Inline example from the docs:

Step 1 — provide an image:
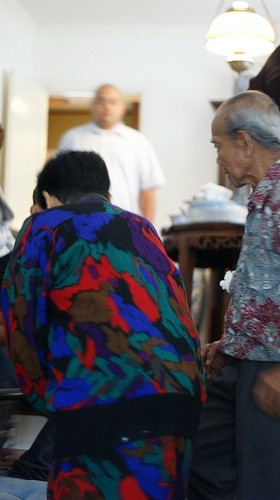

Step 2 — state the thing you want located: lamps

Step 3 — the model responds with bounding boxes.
[204,2,276,74]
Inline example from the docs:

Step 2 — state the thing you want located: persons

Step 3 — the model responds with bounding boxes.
[198,90,279,500]
[56,83,165,224]
[0,187,16,258]
[0,151,208,500]
[30,187,48,215]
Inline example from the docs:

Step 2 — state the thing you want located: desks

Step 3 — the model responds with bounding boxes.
[161,221,243,343]
